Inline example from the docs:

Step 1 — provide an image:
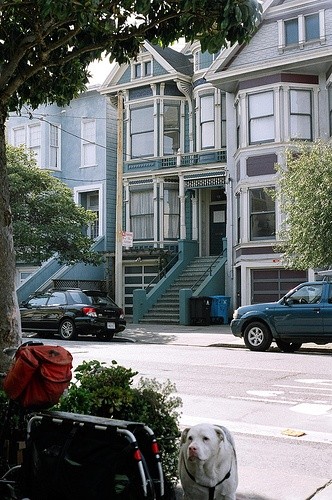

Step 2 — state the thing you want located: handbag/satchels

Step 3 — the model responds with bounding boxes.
[4,343,73,410]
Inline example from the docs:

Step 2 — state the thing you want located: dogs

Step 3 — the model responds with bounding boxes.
[178,424,239,500]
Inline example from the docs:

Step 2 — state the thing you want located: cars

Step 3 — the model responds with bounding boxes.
[230,281,332,353]
[19,287,127,342]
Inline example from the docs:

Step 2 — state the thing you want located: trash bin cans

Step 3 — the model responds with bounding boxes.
[221,237,228,257]
[187,294,232,326]
